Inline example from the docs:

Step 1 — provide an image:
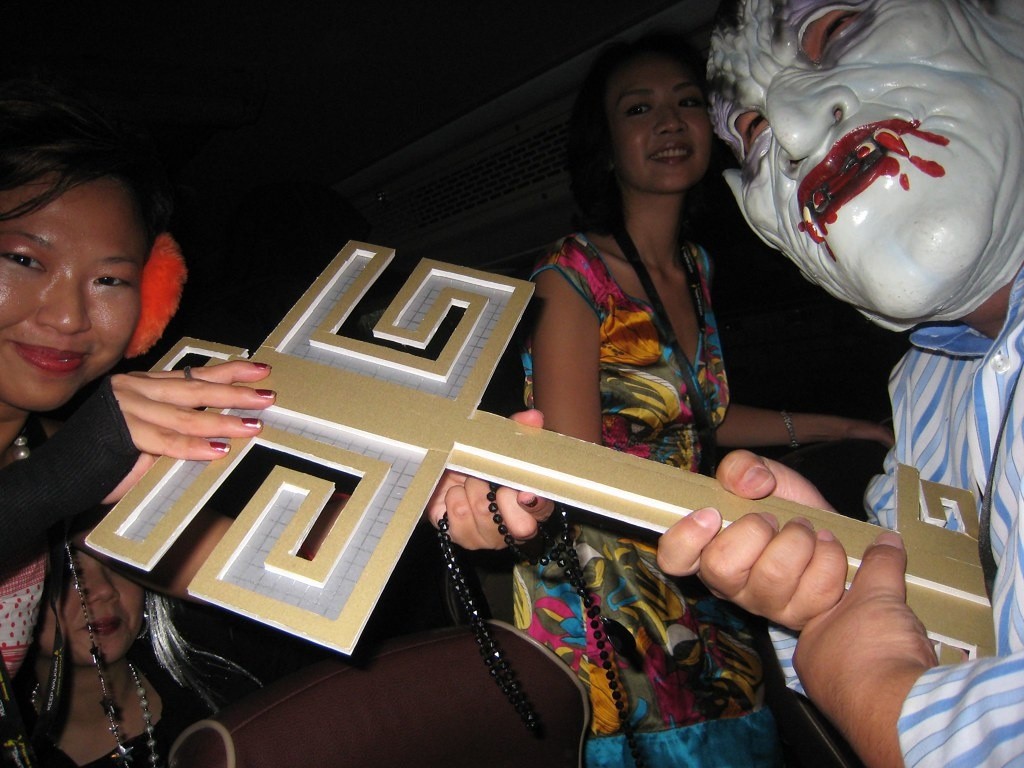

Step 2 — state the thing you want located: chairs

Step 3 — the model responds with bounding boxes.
[169,618,590,768]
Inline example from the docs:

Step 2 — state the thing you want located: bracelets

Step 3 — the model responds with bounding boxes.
[779,411,797,447]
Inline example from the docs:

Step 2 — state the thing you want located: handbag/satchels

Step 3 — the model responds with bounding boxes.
[168,619,591,768]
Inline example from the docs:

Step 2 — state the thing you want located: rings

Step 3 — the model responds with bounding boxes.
[183,365,197,381]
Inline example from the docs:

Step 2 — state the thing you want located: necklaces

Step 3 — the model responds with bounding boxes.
[436,485,646,768]
[13,428,159,767]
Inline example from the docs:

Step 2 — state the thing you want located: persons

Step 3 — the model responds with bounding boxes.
[0,0,1024,768]
